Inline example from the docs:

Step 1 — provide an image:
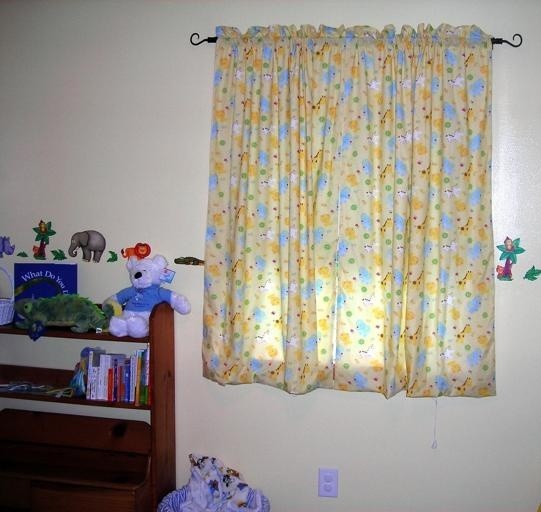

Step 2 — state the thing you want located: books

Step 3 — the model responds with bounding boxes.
[46,342,150,407]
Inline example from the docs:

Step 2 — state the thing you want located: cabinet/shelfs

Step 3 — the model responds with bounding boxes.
[0,301,178,512]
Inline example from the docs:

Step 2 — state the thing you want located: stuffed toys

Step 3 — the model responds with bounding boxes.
[103,255,191,339]
[12,292,123,336]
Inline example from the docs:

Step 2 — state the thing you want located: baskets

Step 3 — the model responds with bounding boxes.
[0,266,16,325]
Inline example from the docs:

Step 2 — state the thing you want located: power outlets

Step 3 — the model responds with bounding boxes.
[318,468,338,498]
